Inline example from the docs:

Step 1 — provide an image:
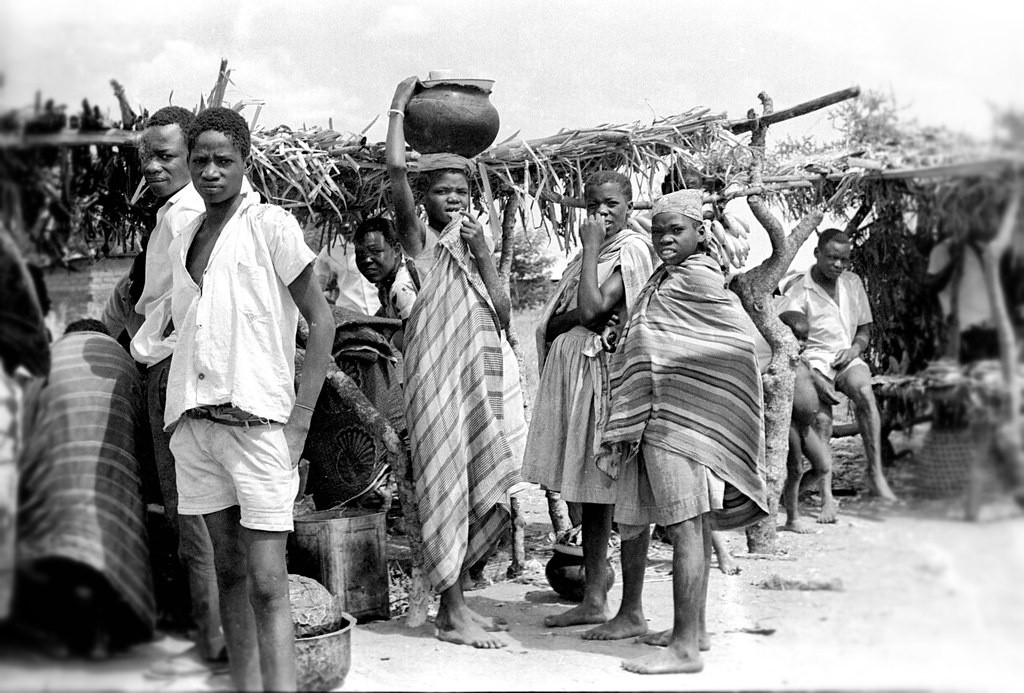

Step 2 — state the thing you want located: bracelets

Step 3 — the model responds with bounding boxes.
[387,109,405,118]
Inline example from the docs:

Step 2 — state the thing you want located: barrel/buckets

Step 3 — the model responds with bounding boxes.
[295,507,392,622]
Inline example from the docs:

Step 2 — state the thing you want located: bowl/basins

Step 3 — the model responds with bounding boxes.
[422,79,495,91]
[555,541,614,560]
[295,612,357,691]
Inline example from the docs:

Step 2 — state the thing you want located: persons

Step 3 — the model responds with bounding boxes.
[0,75,767,693]
[773,229,896,533]
[924,193,1024,365]
